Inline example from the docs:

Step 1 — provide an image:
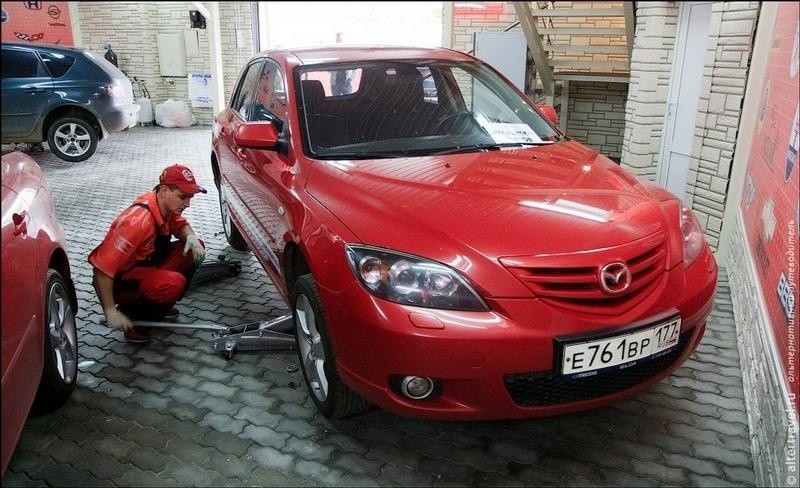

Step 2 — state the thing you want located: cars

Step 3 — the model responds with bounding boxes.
[205,42,720,437]
[416,66,437,96]
[0,41,144,164]
[0,146,83,488]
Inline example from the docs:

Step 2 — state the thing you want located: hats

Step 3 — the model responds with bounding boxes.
[158,162,208,196]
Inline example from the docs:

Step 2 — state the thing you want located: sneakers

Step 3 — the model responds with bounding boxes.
[163,306,181,320]
[123,328,154,343]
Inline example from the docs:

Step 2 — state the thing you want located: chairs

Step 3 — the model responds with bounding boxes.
[296,66,449,148]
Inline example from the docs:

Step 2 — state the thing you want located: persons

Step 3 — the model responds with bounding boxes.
[87,164,209,345]
